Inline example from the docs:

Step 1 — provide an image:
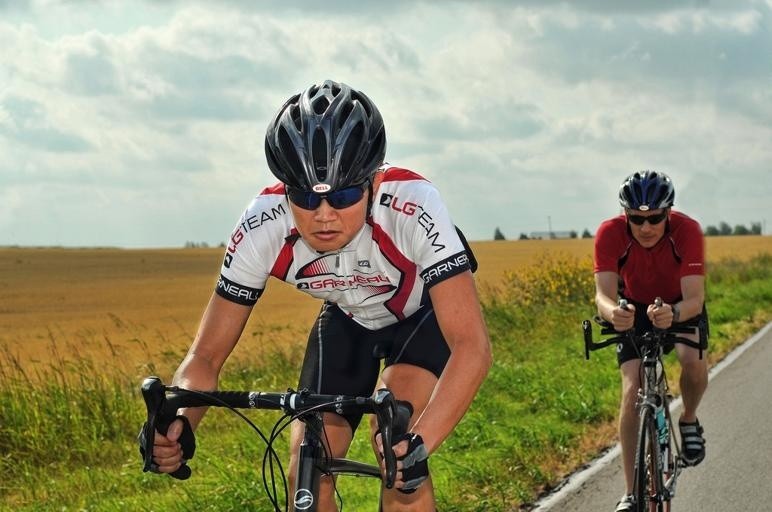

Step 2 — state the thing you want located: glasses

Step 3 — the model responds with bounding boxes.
[282,179,376,210]
[627,208,668,226]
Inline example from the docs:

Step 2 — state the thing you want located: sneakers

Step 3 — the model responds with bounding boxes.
[676,413,707,468]
[612,493,637,511]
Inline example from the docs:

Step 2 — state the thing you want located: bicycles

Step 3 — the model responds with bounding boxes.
[138,373,414,511]
[582,297,705,512]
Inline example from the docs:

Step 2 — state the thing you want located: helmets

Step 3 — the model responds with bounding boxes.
[616,169,676,212]
[263,78,389,195]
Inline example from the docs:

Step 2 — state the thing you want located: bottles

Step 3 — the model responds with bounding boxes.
[657,404,670,444]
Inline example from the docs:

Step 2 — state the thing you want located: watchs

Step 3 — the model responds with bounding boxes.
[670,304,681,320]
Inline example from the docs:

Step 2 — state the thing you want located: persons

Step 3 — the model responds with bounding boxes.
[593,171,709,512]
[139,80,494,511]
